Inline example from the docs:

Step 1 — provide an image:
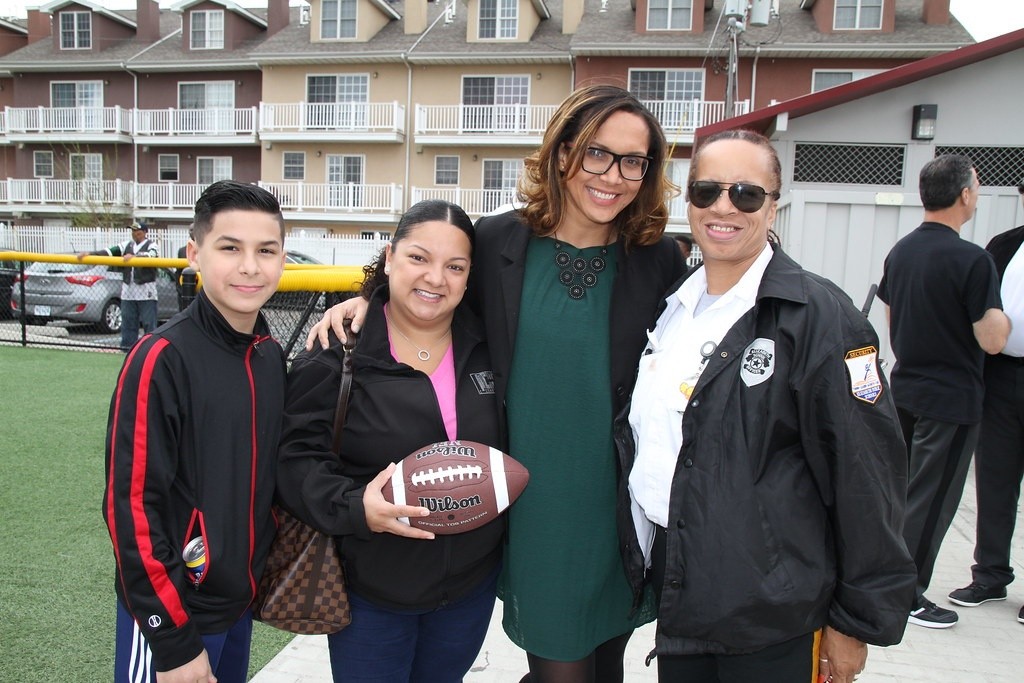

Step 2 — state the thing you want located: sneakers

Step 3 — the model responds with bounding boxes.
[907,595,958,629]
[948,582,1007,606]
[1017,606,1024,623]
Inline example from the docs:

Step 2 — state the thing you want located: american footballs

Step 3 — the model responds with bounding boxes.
[383,440,531,537]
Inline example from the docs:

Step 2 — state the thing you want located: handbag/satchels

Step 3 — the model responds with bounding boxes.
[249,317,351,635]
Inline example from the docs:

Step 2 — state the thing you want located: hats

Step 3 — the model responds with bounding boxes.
[126,222,148,233]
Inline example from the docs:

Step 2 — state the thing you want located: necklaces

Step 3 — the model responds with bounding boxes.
[385,312,452,363]
[548,215,613,301]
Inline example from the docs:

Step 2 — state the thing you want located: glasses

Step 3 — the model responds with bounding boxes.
[687,180,782,213]
[565,142,653,181]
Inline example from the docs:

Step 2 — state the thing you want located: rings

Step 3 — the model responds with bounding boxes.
[819,659,829,663]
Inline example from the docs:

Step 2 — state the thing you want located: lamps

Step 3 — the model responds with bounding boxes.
[473,155,477,160]
[912,104,937,140]
[373,72,377,78]
[105,80,109,84]
[238,80,242,86]
[316,151,321,157]
[537,73,541,79]
[188,153,191,159]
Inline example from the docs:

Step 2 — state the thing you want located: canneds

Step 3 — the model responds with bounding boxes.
[183,536,205,581]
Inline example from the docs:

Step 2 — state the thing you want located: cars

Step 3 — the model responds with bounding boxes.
[176,246,355,312]
[8,250,184,334]
[0,259,37,320]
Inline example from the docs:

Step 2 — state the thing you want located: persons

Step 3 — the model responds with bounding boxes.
[876,154,1012,630]
[300,83,690,683]
[103,181,291,683]
[617,128,918,683]
[948,175,1024,626]
[75,220,161,354]
[173,221,198,317]
[288,202,509,683]
